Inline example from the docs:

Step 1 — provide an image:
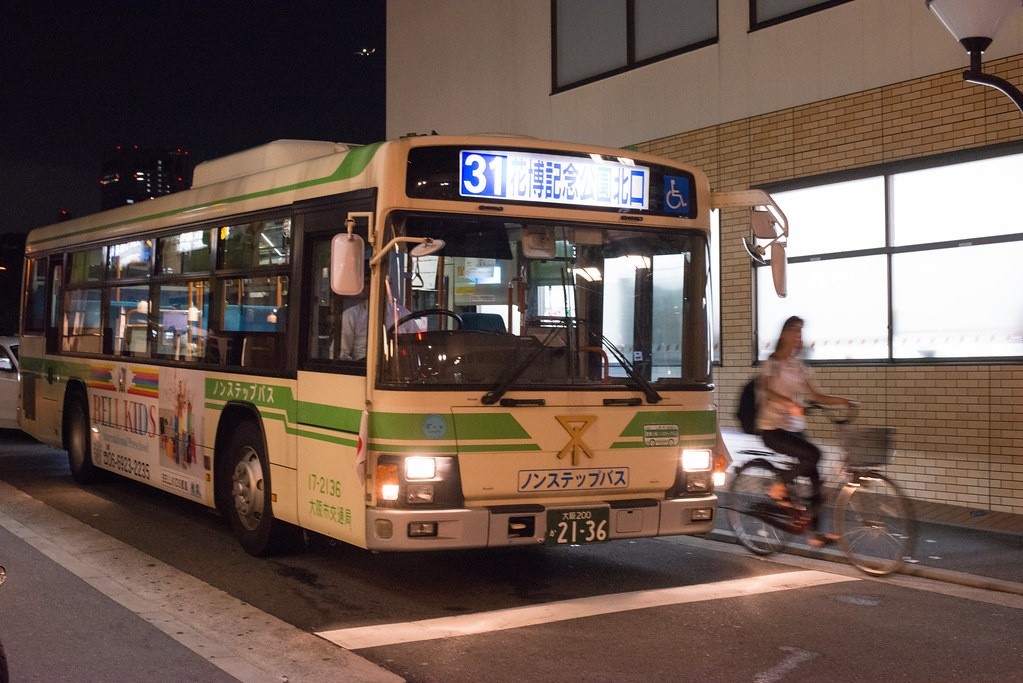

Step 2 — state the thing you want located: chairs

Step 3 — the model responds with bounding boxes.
[458,313,506,333]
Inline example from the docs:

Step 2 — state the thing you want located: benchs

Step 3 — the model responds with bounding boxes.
[206,330,330,370]
[66,327,105,354]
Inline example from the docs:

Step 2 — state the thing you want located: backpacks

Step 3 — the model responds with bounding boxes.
[736,353,783,435]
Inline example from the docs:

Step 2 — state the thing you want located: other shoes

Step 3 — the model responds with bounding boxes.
[808,533,842,548]
[766,487,806,512]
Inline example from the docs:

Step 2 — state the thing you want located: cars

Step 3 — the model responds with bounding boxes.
[0,334,23,429]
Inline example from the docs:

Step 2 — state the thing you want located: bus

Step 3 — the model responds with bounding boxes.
[16,133,788,558]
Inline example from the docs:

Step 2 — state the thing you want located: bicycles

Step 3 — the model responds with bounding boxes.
[717,400,919,577]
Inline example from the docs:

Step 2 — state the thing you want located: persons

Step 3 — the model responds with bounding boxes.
[755,316,860,546]
[188,336,206,356]
[330,259,422,361]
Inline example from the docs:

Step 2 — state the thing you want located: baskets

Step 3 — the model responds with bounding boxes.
[844,427,897,469]
